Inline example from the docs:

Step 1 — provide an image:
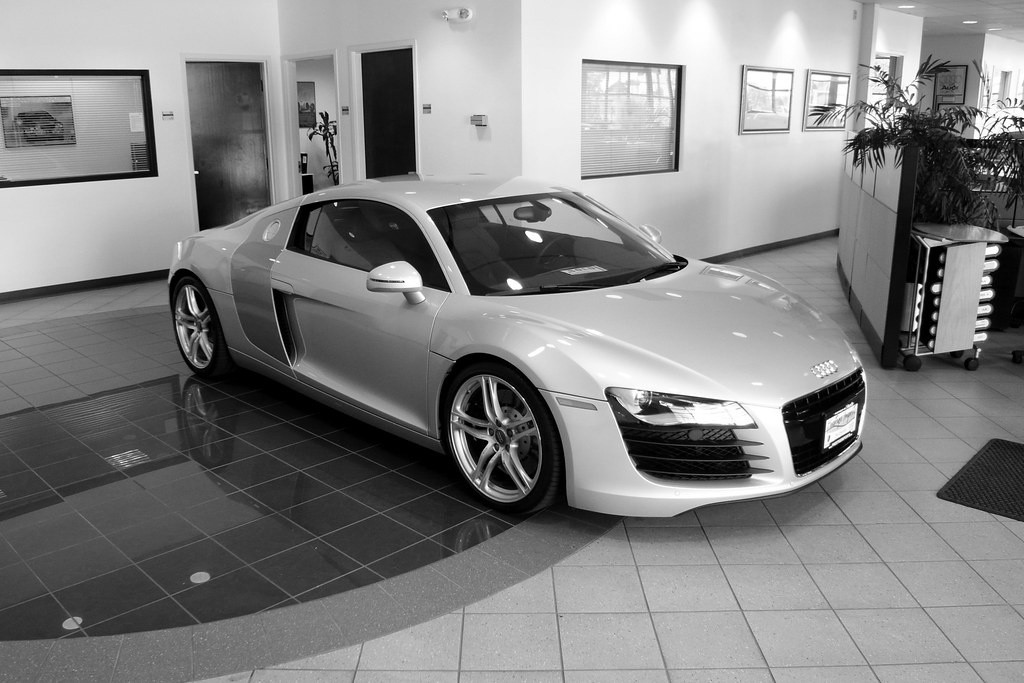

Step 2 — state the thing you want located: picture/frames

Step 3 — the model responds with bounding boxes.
[0,94,76,147]
[802,69,851,132]
[738,64,795,136]
[934,65,968,105]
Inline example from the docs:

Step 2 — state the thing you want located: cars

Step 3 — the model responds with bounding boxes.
[12,111,66,145]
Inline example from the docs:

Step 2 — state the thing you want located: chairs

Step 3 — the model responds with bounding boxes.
[334,206,521,293]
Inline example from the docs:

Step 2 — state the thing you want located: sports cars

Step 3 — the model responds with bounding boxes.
[167,173,867,517]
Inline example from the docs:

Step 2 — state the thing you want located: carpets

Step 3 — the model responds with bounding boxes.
[936,439,1024,521]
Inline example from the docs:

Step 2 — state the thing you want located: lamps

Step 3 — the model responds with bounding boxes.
[441,8,472,21]
